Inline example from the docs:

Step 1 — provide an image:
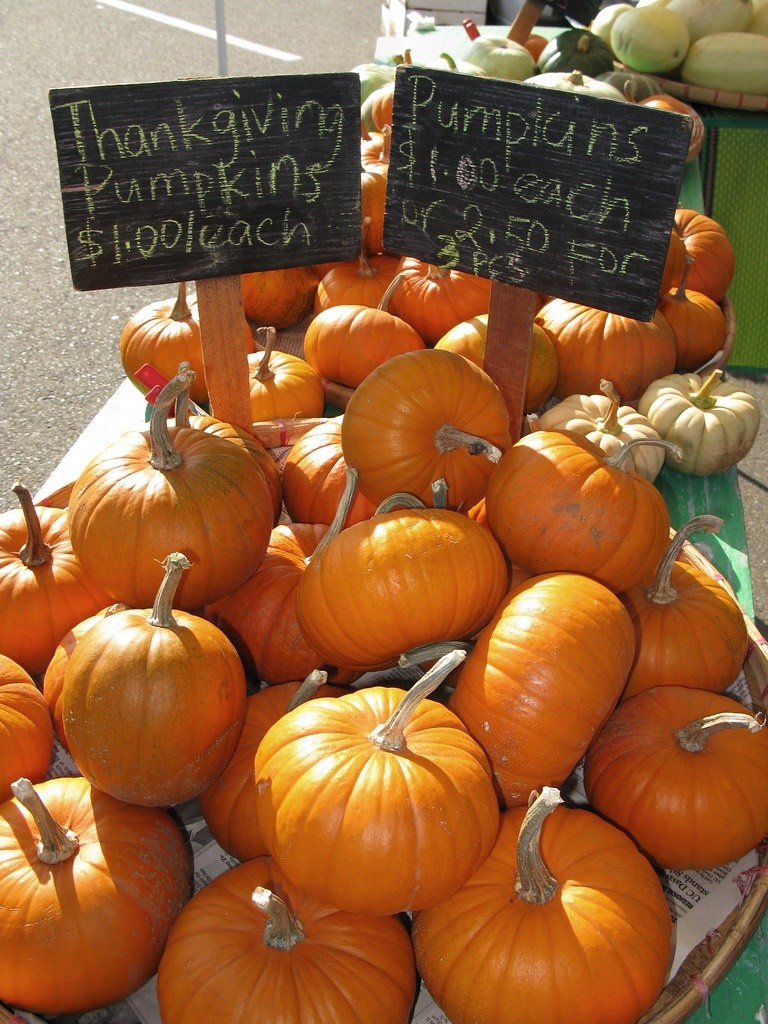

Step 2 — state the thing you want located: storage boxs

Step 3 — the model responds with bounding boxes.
[382,0,487,36]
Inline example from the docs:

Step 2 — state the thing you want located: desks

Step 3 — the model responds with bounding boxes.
[29,26,768,1024]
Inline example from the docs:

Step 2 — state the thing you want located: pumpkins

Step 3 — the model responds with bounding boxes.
[0,0,768,1024]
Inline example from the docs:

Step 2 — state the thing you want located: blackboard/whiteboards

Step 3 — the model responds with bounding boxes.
[380,68,692,323]
[46,73,363,291]
